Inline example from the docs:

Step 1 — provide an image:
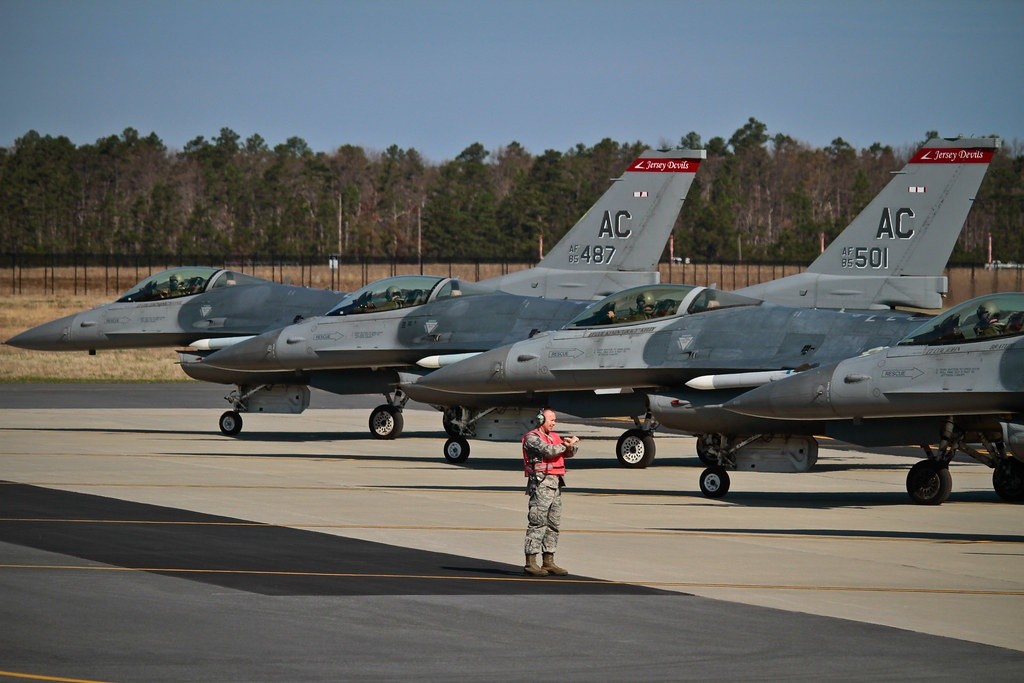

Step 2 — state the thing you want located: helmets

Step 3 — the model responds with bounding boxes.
[169,271,186,290]
[977,301,1001,323]
[385,285,402,302]
[636,292,656,315]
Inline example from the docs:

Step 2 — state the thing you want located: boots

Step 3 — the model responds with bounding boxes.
[541,552,568,576]
[524,553,548,577]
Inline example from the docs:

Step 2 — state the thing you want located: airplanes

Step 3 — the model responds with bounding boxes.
[0,138,1024,507]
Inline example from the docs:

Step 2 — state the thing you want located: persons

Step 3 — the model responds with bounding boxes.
[138,273,190,298]
[352,286,404,313]
[970,300,1006,339]
[522,407,580,577]
[606,291,658,323]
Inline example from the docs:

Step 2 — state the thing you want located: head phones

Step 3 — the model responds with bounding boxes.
[536,408,546,425]
